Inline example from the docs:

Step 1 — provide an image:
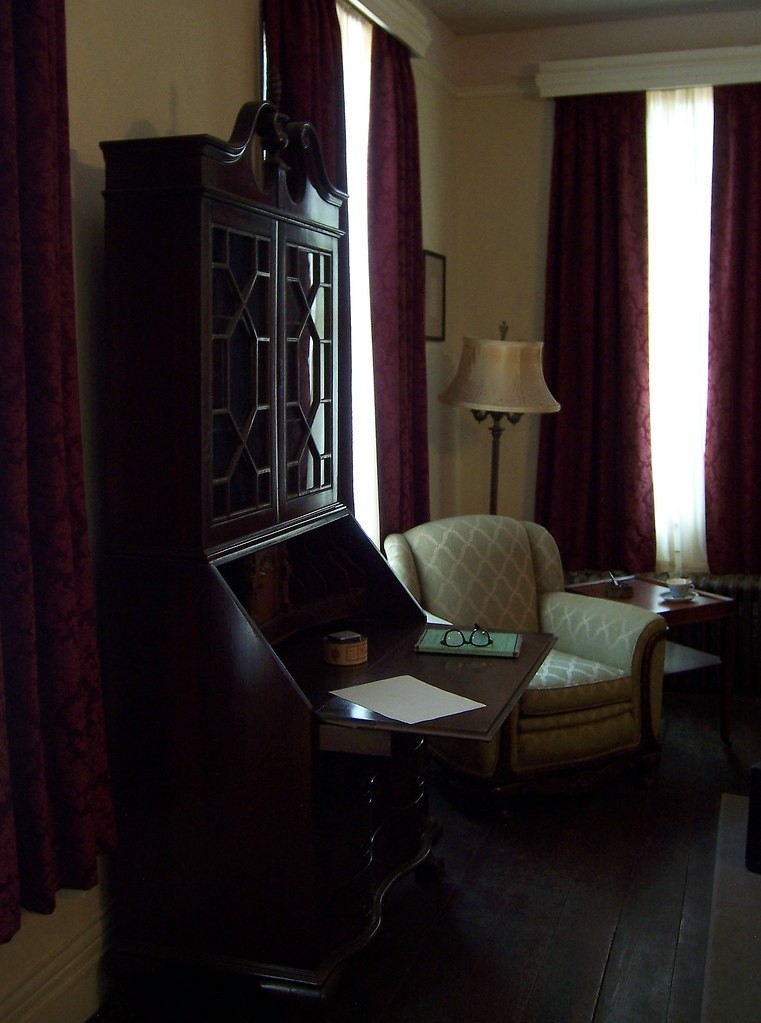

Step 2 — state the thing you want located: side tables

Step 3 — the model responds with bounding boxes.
[566,576,737,751]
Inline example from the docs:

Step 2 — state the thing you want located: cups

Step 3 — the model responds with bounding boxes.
[667,578,694,598]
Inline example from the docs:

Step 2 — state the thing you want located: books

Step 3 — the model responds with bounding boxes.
[414,629,524,657]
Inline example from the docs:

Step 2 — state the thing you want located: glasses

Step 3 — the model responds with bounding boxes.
[440,623,494,646]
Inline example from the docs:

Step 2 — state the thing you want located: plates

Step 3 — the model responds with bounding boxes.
[661,591,698,602]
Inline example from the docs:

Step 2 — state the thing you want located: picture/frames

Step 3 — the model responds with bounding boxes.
[417,249,446,340]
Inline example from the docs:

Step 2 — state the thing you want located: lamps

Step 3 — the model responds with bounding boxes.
[439,317,561,514]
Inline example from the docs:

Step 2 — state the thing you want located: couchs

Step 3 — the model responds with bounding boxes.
[380,513,673,846]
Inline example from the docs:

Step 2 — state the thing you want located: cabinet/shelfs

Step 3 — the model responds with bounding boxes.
[98,101,556,1023]
